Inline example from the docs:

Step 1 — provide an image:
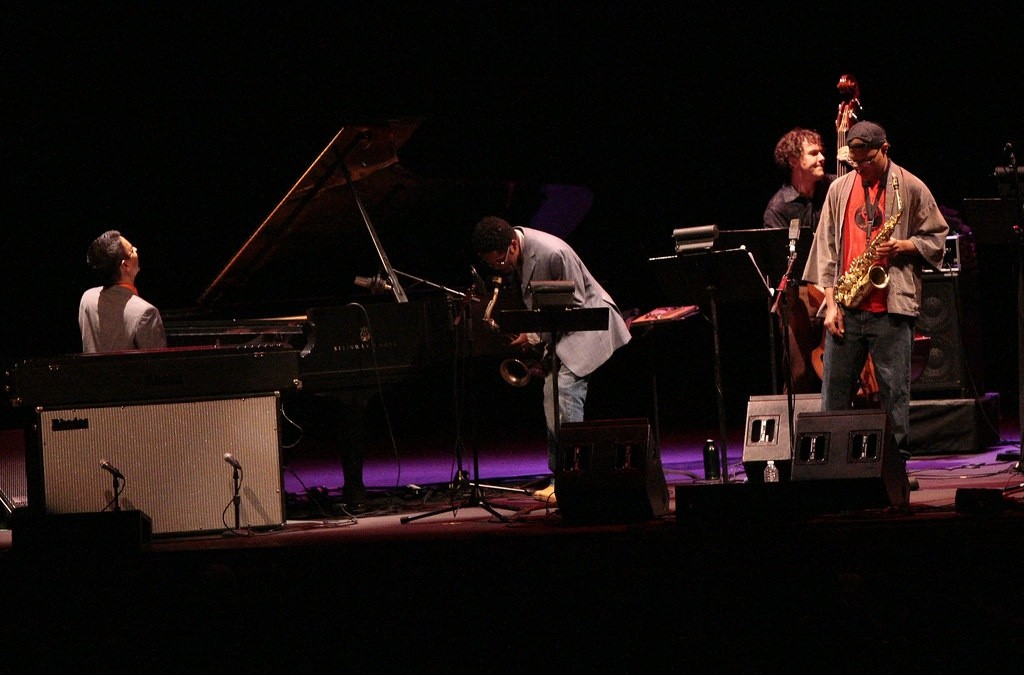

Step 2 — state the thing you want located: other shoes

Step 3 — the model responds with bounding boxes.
[533,482,555,504]
[907,474,920,491]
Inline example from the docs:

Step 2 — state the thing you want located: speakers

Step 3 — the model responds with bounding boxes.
[554,418,670,521]
[908,272,964,391]
[25,390,286,535]
[740,393,910,511]
[12,510,154,549]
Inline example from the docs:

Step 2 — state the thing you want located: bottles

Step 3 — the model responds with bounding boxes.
[764,460,780,482]
[703,439,722,480]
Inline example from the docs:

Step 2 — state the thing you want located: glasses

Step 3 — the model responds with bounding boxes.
[849,143,883,168]
[485,244,511,268]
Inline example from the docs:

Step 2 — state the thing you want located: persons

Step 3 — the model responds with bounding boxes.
[801,122,950,490]
[472,215,632,502]
[764,130,837,394]
[76,230,168,352]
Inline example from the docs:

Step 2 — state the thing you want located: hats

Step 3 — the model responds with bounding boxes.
[846,121,891,148]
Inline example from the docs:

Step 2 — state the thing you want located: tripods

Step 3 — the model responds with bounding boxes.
[381,267,534,525]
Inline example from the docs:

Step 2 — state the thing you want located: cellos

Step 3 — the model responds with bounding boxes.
[809,70,932,398]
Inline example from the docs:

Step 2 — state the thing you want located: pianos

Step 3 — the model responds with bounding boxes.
[3,342,303,512]
[155,124,459,510]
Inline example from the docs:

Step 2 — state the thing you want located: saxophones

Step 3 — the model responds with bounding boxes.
[481,270,548,389]
[833,175,906,308]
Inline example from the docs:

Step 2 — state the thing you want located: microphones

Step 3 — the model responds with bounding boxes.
[223,453,241,469]
[99,459,124,480]
[354,276,392,290]
[789,218,802,252]
[1006,144,1016,164]
